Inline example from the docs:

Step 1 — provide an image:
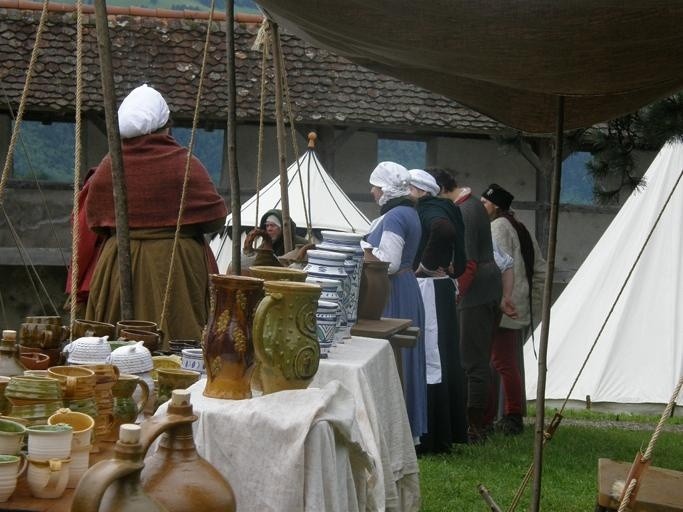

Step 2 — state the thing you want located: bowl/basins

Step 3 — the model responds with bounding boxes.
[16,315,163,348]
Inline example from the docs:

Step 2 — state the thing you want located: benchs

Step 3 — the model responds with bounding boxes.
[598,458,683,512]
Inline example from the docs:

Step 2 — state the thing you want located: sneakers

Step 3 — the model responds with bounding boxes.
[503,413,524,436]
[467,426,487,445]
[493,418,505,432]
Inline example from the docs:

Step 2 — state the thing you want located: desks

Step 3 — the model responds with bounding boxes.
[3,313,389,510]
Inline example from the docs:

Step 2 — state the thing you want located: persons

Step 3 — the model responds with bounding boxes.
[429,172,502,444]
[243,210,309,267]
[88,86,228,346]
[479,185,531,434]
[360,160,424,455]
[405,170,464,456]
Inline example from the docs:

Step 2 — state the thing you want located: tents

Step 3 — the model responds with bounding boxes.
[520,133,683,407]
[208,131,377,282]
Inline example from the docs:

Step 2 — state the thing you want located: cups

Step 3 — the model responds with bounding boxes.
[1,363,150,512]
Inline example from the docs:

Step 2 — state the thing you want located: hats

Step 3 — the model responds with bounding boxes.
[481,183,514,211]
